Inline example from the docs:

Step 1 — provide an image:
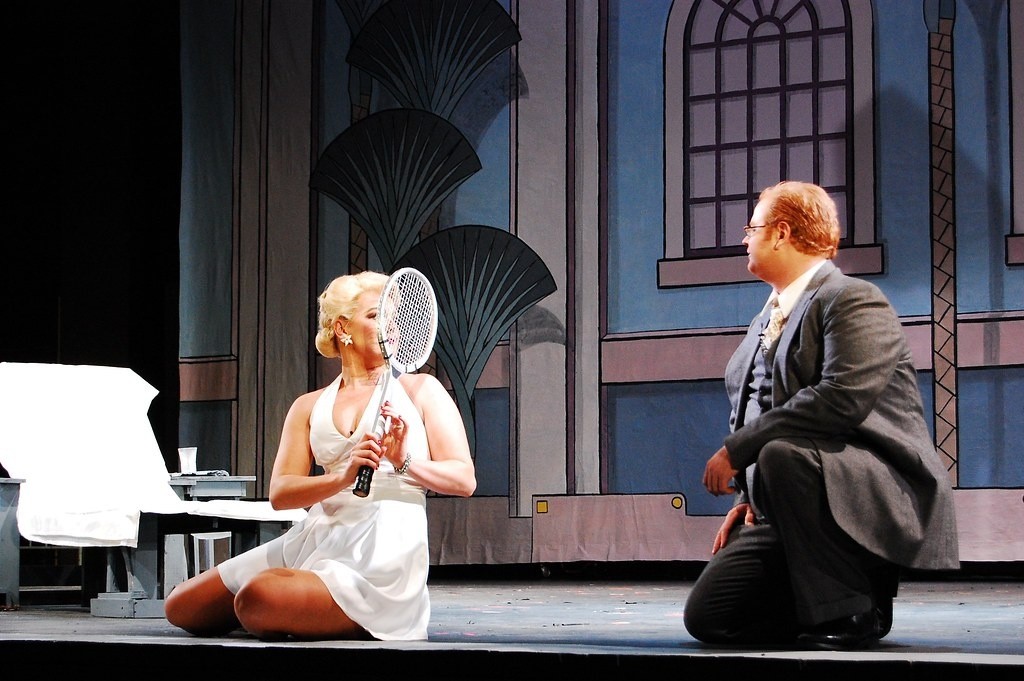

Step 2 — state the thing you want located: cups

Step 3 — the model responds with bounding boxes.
[178,447,197,474]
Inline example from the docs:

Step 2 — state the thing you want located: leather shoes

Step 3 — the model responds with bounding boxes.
[851,567,899,640]
[799,608,877,649]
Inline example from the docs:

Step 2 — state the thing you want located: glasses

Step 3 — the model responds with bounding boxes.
[744,225,765,237]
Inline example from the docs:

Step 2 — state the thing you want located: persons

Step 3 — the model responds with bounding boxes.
[165,271,476,640]
[683,181,961,650]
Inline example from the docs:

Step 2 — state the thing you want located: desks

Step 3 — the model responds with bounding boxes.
[172,472,257,575]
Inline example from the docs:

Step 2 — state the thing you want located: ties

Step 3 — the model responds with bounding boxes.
[761,308,784,349]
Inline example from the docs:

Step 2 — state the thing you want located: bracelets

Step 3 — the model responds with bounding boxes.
[394,452,412,475]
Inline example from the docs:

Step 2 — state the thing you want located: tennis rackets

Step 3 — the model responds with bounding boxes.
[353,265,438,500]
